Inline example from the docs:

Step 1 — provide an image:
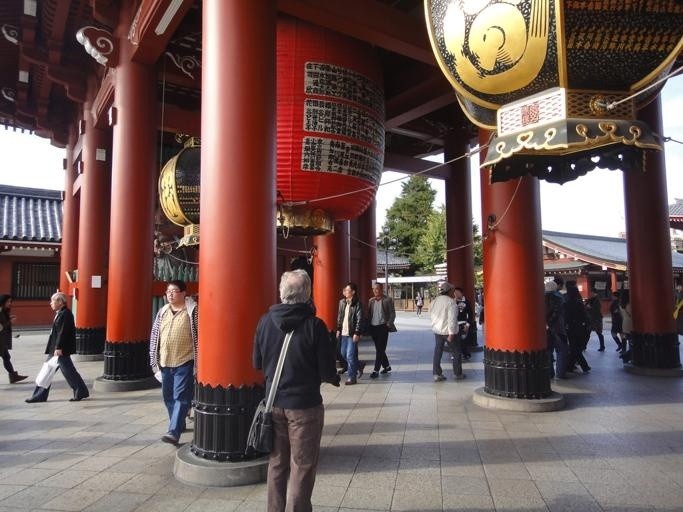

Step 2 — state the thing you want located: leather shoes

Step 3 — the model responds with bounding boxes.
[358,361,366,377]
[70,394,89,401]
[26,399,45,402]
[345,378,356,385]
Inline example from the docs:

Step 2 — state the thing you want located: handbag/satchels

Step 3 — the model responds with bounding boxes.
[245,399,272,455]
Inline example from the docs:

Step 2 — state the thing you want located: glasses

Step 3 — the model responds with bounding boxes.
[166,290,181,294]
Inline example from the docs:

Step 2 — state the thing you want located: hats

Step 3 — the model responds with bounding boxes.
[441,283,454,291]
[545,281,557,292]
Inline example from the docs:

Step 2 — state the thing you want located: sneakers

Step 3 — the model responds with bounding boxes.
[381,367,391,373]
[454,374,466,379]
[162,434,177,444]
[434,374,446,381]
[370,372,378,377]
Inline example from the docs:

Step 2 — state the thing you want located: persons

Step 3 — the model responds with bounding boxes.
[337,287,358,374]
[25,292,89,403]
[0,295,28,383]
[428,283,467,381]
[415,291,424,318]
[335,283,366,385]
[451,287,473,360]
[475,279,683,378]
[149,280,198,445]
[366,283,397,377]
[252,268,341,512]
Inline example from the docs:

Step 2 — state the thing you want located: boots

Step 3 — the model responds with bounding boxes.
[614,336,623,351]
[9,371,27,383]
[619,339,626,353]
[598,335,605,352]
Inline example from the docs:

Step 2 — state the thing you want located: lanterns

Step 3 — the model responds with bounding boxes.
[157,137,201,250]
[276,14,385,236]
[424,0,683,186]
